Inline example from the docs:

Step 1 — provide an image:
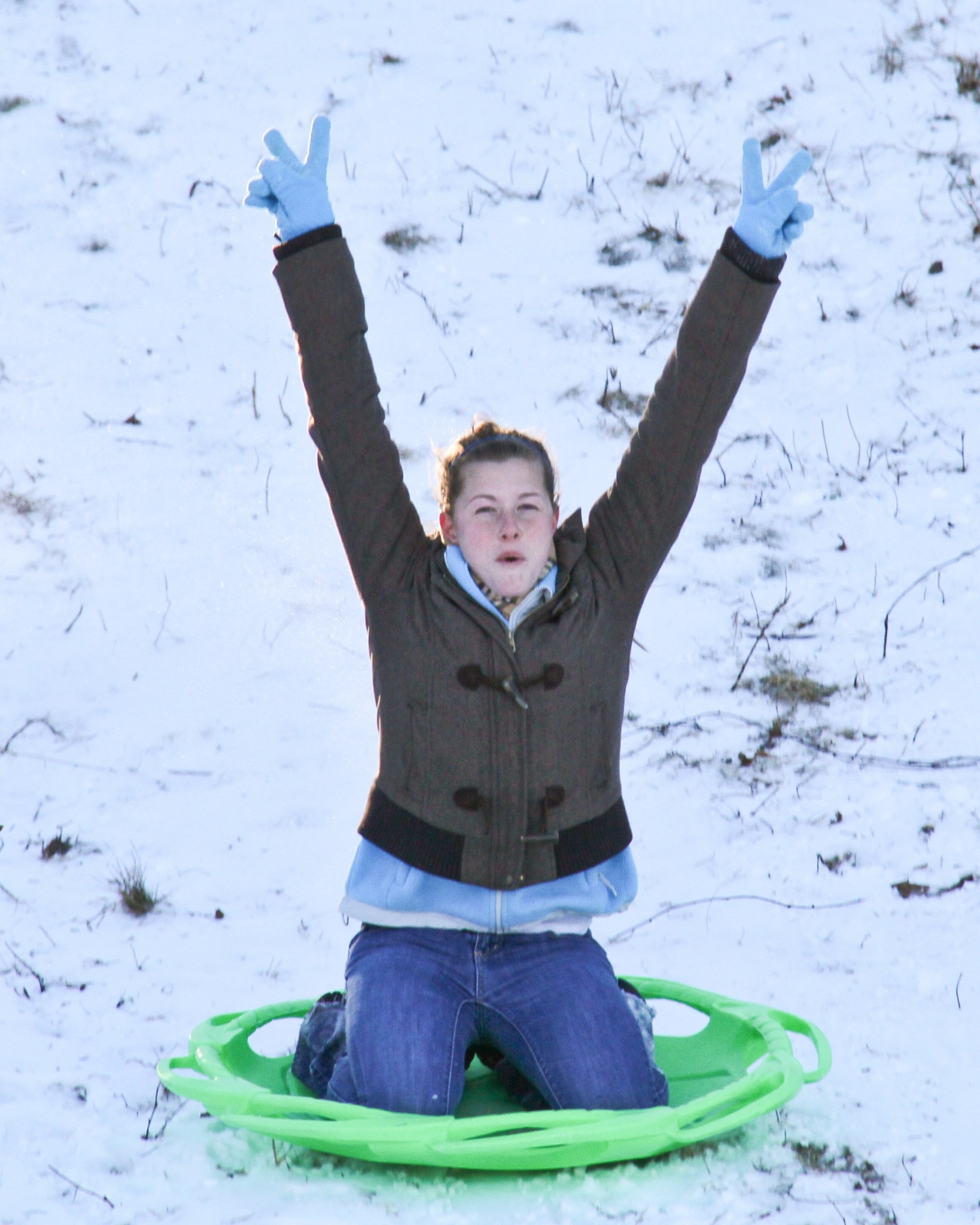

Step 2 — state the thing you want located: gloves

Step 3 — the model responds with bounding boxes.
[242,113,337,239]
[724,135,816,256]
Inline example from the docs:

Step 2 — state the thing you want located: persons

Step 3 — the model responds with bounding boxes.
[242,114,814,1109]
